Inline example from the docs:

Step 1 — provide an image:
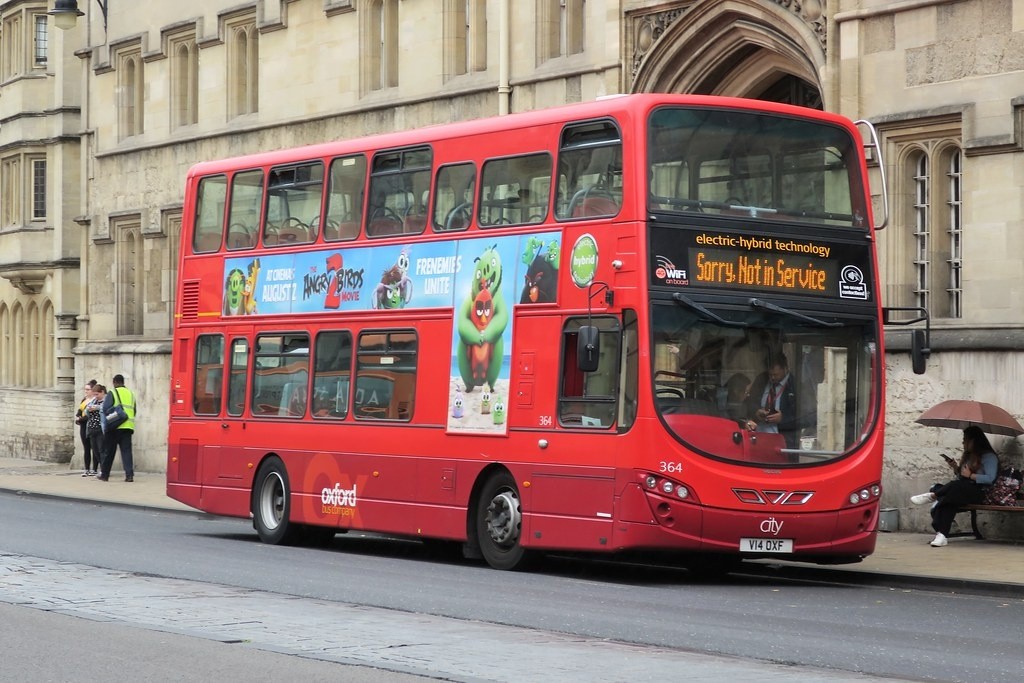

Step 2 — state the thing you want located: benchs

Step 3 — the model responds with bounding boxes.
[949,469,1024,540]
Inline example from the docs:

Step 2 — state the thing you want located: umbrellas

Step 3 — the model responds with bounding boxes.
[915,399,1024,465]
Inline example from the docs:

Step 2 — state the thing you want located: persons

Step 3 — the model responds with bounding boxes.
[911,425,999,547]
[97,374,137,482]
[76,380,108,478]
[703,353,800,464]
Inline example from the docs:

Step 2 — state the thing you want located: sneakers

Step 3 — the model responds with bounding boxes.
[931,532,948,546]
[911,492,935,505]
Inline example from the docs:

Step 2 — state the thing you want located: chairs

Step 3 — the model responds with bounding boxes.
[720,197,748,216]
[196,183,619,251]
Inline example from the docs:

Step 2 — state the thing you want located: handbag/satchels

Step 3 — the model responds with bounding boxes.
[991,467,1024,506]
[105,405,128,431]
[974,484,991,505]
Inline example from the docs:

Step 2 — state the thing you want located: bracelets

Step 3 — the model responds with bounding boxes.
[969,472,973,479]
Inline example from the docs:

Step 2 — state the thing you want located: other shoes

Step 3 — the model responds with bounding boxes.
[88,472,98,475]
[82,470,89,476]
[125,476,134,482]
[96,476,108,482]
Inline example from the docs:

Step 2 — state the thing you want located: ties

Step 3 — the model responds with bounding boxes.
[766,383,778,409]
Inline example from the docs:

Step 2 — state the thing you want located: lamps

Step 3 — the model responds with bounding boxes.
[46,0,107,33]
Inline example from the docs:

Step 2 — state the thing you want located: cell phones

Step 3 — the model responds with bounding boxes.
[940,454,952,462]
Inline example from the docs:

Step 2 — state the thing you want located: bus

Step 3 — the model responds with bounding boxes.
[167,93,935,579]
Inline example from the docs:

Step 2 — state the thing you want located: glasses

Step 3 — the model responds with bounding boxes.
[85,388,92,391]
[744,391,750,395]
